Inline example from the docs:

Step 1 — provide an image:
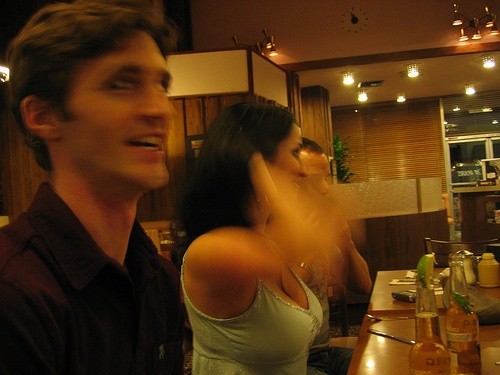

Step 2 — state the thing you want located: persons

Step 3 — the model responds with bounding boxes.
[292,137,372,375]
[0,0,184,375]
[180,103,323,375]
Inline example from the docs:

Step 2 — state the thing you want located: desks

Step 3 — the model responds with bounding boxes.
[349,269,500,375]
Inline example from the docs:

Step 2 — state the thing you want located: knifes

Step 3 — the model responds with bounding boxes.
[367,328,416,345]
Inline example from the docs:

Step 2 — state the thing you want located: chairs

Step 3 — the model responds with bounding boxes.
[425,236,498,267]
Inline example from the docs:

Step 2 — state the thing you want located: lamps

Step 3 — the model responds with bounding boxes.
[231,28,277,57]
[357,90,368,102]
[481,56,495,68]
[407,64,419,77]
[452,3,499,42]
[343,71,354,85]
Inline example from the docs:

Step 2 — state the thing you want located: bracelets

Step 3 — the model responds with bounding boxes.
[297,261,314,285]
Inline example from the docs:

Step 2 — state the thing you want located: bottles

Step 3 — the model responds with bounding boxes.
[455,249,476,285]
[446,255,481,375]
[477,252,500,288]
[409,275,450,375]
